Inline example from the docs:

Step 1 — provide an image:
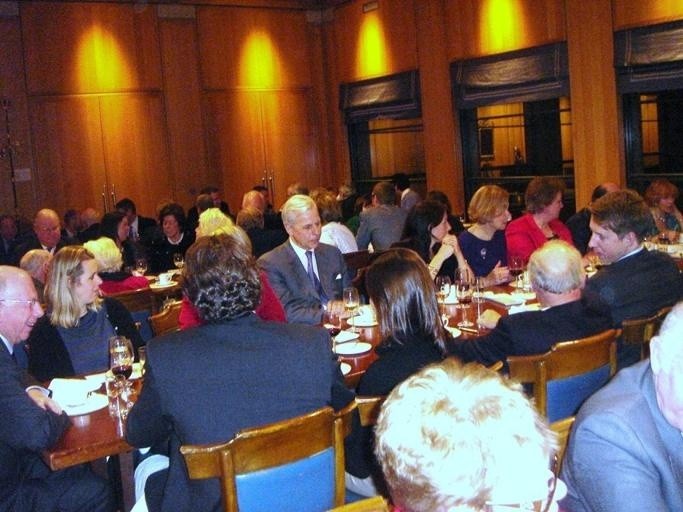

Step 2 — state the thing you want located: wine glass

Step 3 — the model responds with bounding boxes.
[505,253,527,296]
[133,254,185,276]
[326,288,360,341]
[107,334,137,412]
[431,269,488,334]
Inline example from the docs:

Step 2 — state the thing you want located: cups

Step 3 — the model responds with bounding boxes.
[104,375,129,420]
[158,274,172,285]
[137,346,148,366]
[356,304,373,321]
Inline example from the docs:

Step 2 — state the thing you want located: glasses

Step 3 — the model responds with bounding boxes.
[0,298,34,305]
[485,453,558,511]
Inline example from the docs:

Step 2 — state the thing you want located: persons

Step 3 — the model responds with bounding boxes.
[1,174,683,512]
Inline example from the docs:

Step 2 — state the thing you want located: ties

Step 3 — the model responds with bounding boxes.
[130,226,132,232]
[47,247,52,252]
[304,251,321,296]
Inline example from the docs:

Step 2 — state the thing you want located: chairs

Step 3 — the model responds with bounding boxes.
[17,228,683,512]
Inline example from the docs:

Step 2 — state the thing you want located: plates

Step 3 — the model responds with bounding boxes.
[339,362,351,376]
[54,393,110,418]
[148,281,179,288]
[332,339,372,355]
[346,315,378,327]
